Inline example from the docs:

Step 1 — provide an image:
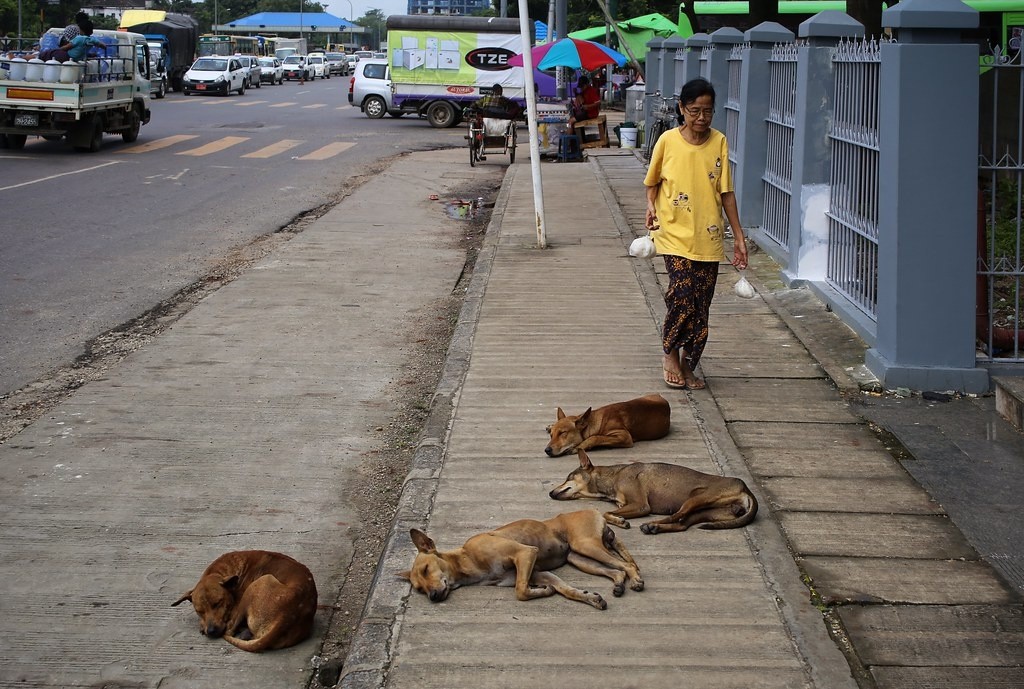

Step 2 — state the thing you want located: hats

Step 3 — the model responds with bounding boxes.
[577,76,590,88]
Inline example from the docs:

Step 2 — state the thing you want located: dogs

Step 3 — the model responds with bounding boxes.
[550,449,758,534]
[545,393,671,457]
[171,550,318,653]
[393,509,644,610]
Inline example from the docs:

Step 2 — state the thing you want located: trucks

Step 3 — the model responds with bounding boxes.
[0,28,167,154]
[119,11,196,93]
[384,12,590,128]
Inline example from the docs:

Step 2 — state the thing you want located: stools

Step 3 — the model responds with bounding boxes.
[558,135,582,163]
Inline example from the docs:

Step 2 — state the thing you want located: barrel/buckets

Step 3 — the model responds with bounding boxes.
[0,52,133,84]
[619,128,638,149]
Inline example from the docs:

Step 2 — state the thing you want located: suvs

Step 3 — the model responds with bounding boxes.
[347,58,421,119]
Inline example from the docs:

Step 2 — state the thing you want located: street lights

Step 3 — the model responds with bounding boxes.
[218,8,230,24]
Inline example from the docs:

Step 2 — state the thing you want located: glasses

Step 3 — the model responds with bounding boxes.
[681,102,715,116]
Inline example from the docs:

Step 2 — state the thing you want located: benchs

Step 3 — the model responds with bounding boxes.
[574,115,611,151]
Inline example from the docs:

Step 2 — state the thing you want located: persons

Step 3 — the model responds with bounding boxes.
[7,20,106,63]
[642,79,749,391]
[472,83,518,128]
[60,11,89,47]
[573,88,579,98]
[32,44,40,51]
[298,57,304,85]
[569,76,601,135]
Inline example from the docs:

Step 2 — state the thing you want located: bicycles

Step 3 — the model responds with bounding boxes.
[644,89,681,166]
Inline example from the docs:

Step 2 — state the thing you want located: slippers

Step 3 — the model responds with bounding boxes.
[678,361,705,390]
[662,357,686,387]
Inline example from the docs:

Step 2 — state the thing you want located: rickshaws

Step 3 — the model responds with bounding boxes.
[461,107,518,167]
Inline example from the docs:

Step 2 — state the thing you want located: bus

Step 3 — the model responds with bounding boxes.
[196,33,259,60]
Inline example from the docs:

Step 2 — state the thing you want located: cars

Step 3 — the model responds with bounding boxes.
[243,36,387,89]
[182,56,246,96]
[146,60,168,98]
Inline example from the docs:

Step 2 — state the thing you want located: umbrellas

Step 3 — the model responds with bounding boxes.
[506,37,629,101]
